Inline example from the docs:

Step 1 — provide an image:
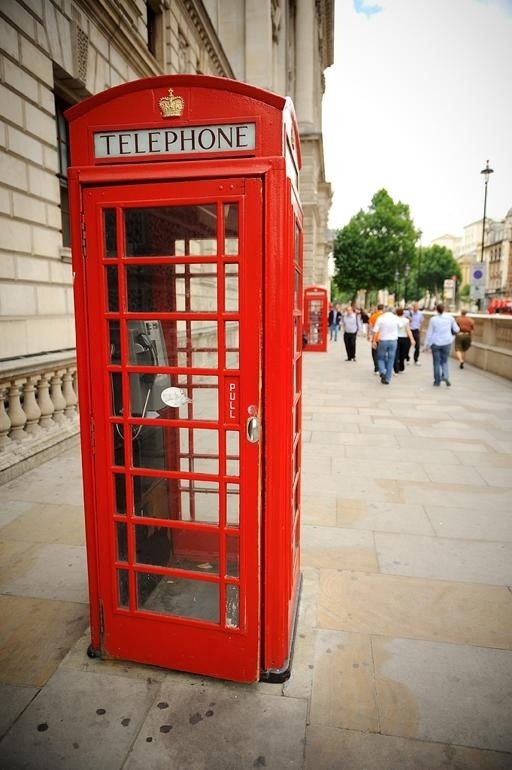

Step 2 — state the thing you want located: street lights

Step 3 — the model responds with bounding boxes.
[394,265,409,309]
[415,229,423,301]
[480,161,494,264]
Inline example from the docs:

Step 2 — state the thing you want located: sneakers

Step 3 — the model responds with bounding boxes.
[329,337,464,386]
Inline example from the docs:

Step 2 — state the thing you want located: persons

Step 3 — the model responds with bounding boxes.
[309,301,475,386]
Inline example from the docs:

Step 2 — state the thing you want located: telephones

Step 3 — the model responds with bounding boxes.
[110,320,172,415]
